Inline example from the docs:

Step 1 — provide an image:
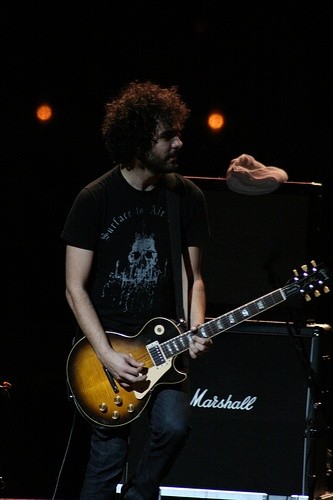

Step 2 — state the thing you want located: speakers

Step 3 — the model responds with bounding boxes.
[158,319,323,496]
[179,176,325,318]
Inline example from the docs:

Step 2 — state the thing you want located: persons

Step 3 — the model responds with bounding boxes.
[51,81,212,500]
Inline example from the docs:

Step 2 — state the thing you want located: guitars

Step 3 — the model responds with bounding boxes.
[64,259,331,430]
